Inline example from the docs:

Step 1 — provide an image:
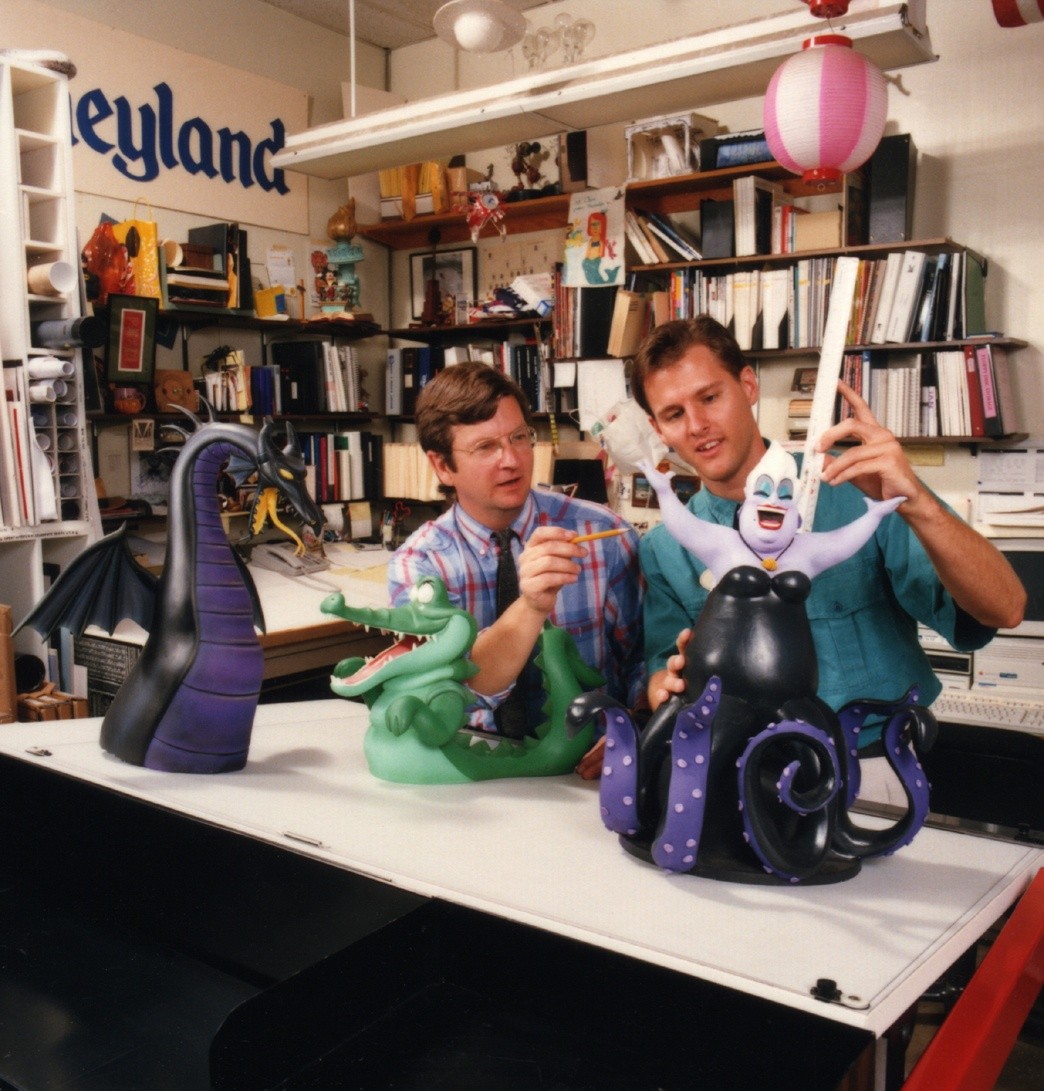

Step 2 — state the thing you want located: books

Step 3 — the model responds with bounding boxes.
[623,165,867,265]
[606,247,985,357]
[782,343,1006,440]
[200,336,361,414]
[0,357,42,531]
[918,492,1044,706]
[161,221,257,318]
[268,427,378,503]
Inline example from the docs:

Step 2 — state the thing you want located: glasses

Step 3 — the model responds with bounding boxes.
[447,426,537,457]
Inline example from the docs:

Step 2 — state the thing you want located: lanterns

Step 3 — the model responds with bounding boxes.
[762,33,889,186]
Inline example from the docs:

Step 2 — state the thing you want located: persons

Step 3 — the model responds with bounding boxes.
[386,357,639,781]
[626,314,1027,814]
[632,438,912,713]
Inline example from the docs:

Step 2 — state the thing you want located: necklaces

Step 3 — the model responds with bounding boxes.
[738,531,795,572]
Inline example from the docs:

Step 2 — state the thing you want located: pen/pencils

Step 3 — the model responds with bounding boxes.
[571,528,631,543]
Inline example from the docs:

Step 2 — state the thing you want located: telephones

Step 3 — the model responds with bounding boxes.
[250,541,329,576]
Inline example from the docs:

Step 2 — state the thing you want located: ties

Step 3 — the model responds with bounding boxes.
[491,530,536,737]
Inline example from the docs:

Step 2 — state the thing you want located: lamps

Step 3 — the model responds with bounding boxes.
[431,0,528,50]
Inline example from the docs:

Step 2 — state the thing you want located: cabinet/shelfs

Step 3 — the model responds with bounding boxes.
[343,133,1044,751]
[93,285,377,553]
[1,49,103,723]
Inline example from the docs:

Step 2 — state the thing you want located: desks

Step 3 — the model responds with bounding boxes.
[77,545,399,717]
